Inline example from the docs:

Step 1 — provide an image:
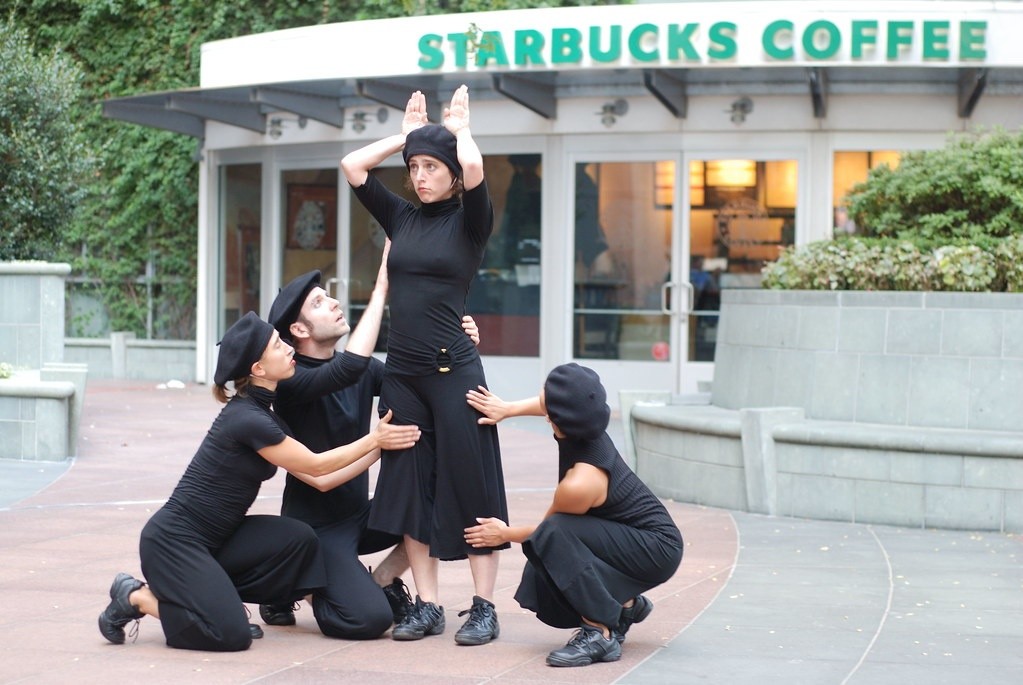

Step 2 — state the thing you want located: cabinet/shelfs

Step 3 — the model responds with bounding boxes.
[710,212,795,272]
[575,280,627,357]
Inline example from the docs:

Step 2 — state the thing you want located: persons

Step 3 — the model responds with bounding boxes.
[97,312,422,653]
[268,233,480,640]
[580,243,620,319]
[665,254,720,310]
[341,85,511,648]
[464,364,683,668]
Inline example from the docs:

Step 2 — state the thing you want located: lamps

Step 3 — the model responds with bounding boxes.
[601,98,628,126]
[270,116,308,137]
[351,106,388,131]
[729,96,753,122]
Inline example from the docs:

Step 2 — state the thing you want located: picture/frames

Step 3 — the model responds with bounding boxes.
[285,183,337,249]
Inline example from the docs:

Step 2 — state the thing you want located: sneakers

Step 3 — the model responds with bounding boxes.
[259,600,301,625]
[392,595,446,639]
[615,594,653,643]
[455,595,500,645]
[98,572,147,644]
[382,577,416,624]
[546,620,621,667]
[242,604,264,639]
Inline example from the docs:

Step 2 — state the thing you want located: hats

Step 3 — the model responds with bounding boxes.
[214,311,275,386]
[544,362,610,440]
[268,270,324,341]
[402,124,462,181]
